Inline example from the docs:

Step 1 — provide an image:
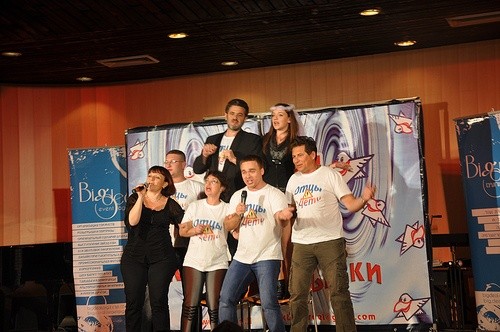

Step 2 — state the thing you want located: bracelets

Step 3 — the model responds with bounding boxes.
[360,195,367,204]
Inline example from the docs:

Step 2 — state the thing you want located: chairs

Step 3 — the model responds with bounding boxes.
[247,286,319,332]
[200,287,250,332]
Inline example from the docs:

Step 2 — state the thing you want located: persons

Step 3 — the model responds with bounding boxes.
[219,154,294,332]
[180,170,239,332]
[119,165,190,332]
[282,135,376,332]
[165,99,306,301]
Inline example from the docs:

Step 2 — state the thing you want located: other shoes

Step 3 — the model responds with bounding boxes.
[276,279,288,300]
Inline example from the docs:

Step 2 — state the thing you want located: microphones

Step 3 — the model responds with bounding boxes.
[132,183,149,194]
[240,191,247,219]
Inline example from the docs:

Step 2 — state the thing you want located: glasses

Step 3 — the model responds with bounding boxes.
[164,159,184,164]
